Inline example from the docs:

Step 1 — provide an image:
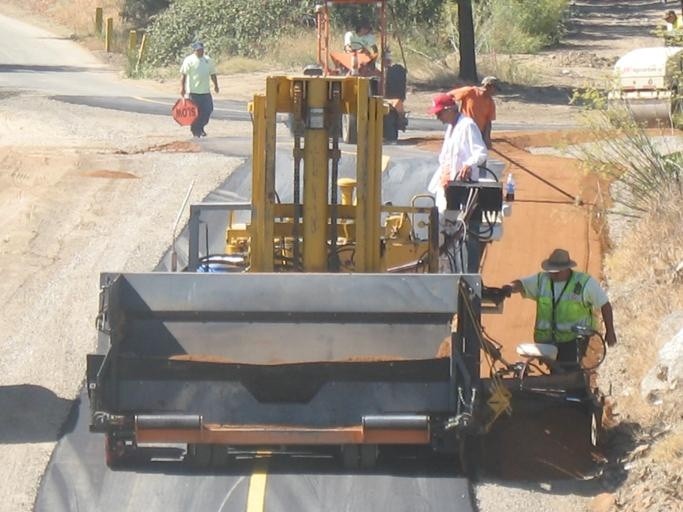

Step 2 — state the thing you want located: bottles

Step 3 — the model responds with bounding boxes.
[481,75,497,86]
[426,93,456,114]
[541,248,578,272]
[191,42,203,50]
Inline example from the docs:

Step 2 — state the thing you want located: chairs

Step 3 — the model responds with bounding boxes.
[200,129,206,136]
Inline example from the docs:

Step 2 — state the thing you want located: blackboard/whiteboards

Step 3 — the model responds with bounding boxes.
[286,0,408,144]
[85,74,510,466]
[603,44,683,129]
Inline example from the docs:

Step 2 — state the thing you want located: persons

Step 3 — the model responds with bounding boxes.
[178,42,219,138]
[448,77,502,180]
[344,23,378,75]
[502,249,617,370]
[428,94,487,245]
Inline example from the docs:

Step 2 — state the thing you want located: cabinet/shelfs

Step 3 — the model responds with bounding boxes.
[505,173,515,202]
